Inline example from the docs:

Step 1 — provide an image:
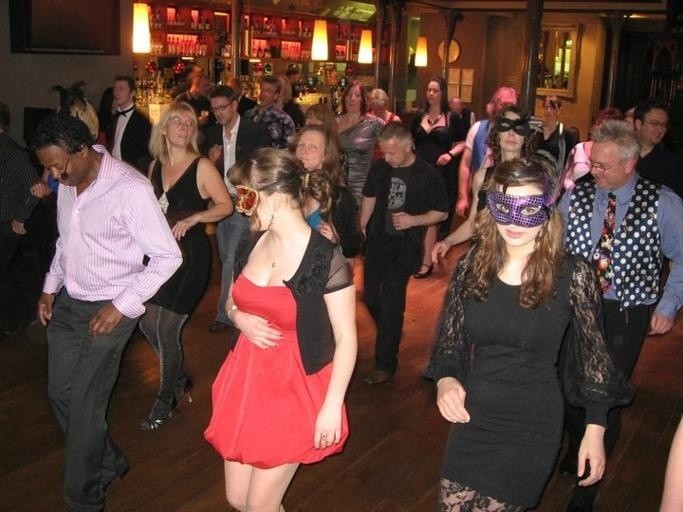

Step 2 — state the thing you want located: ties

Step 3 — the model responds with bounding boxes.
[592,193,616,295]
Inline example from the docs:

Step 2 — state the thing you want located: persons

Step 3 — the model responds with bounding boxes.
[0,60,682,429]
[658,416,682,512]
[550,118,682,512]
[204,148,358,512]
[30,114,182,512]
[418,154,633,512]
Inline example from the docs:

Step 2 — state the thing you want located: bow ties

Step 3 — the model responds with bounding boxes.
[116,110,126,118]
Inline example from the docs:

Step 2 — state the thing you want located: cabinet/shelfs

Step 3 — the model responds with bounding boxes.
[118,0,236,106]
[235,0,376,105]
[6,1,121,59]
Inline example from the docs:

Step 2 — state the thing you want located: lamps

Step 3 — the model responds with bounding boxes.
[413,0,428,67]
[311,0,328,61]
[356,1,374,64]
[130,1,152,53]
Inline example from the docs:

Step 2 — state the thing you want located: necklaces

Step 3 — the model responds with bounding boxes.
[270,258,279,268]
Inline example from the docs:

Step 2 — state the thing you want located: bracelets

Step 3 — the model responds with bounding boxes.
[224,304,236,323]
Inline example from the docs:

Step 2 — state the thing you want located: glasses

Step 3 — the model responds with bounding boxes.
[212,101,232,112]
[585,159,623,172]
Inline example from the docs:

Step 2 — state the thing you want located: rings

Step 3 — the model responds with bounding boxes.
[322,434,325,440]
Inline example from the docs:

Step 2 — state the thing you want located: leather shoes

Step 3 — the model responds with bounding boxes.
[415,261,434,278]
[209,321,228,332]
[364,369,392,383]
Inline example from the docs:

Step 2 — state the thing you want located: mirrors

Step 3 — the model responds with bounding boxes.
[520,21,581,100]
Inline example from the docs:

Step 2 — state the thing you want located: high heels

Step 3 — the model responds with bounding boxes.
[170,376,193,416]
[139,396,176,432]
